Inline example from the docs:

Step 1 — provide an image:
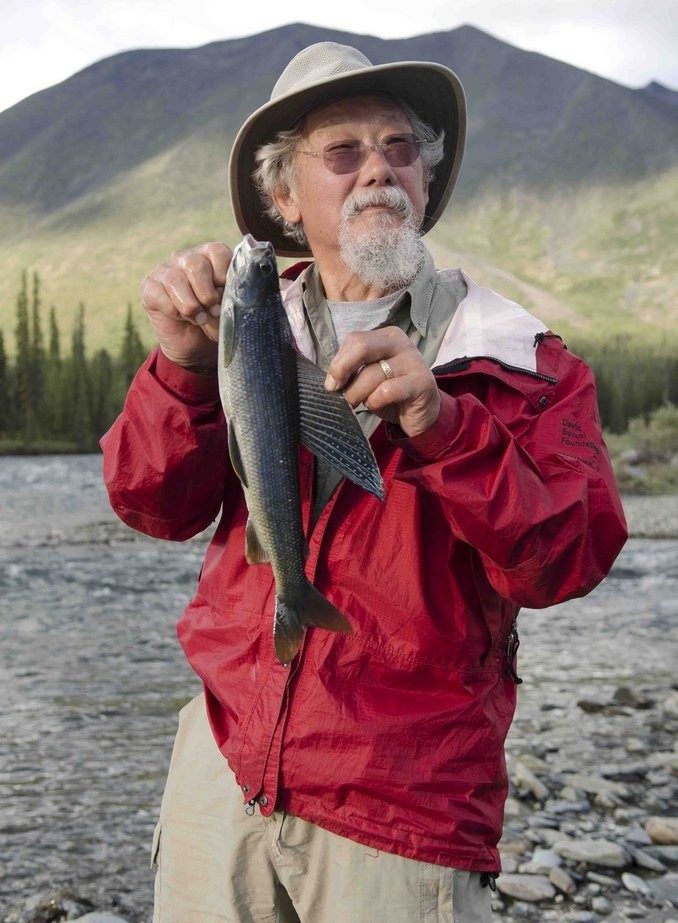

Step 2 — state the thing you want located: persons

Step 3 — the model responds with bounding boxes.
[98,42,629,923]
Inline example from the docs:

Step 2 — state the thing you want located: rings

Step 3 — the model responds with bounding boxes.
[378,359,394,380]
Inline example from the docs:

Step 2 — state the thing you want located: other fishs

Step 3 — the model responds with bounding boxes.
[216,234,386,669]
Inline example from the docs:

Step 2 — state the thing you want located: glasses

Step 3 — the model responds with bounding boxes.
[288,133,425,175]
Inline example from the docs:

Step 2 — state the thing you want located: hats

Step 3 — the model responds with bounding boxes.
[230,41,467,258]
